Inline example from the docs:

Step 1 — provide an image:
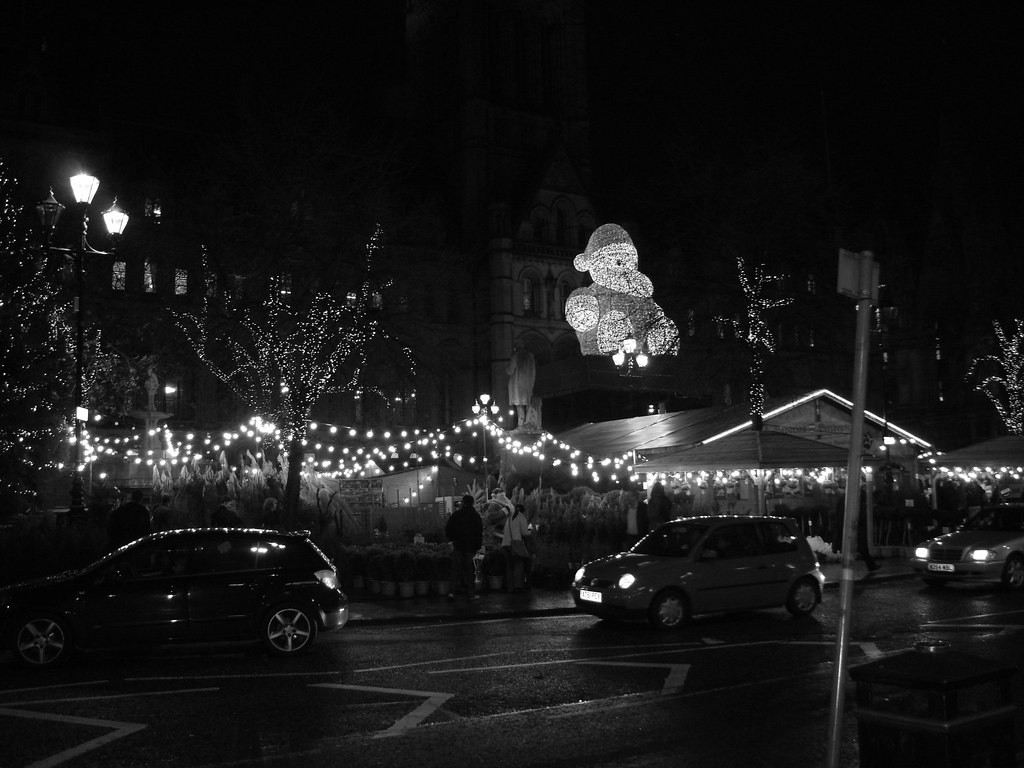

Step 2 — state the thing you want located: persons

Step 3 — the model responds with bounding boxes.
[647,482,673,529]
[620,491,648,551]
[445,495,482,600]
[211,498,242,528]
[262,497,279,530]
[503,503,537,596]
[111,489,151,547]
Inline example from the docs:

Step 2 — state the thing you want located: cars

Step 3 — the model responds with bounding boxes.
[570,515,824,633]
[908,503,1024,600]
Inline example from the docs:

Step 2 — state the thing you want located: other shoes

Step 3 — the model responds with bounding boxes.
[515,589,524,594]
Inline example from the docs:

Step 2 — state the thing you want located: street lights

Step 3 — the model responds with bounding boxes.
[36,167,130,511]
[471,391,500,488]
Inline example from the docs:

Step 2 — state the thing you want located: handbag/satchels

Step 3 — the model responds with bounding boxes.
[511,540,531,560]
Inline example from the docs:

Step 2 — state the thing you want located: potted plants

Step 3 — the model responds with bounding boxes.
[873,488,940,558]
[348,515,505,598]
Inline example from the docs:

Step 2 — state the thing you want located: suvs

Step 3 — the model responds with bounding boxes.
[0,528,349,681]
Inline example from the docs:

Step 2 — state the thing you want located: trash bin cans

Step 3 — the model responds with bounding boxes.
[847,639,1024,768]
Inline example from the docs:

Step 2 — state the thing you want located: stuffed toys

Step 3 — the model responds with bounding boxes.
[485,487,514,590]
